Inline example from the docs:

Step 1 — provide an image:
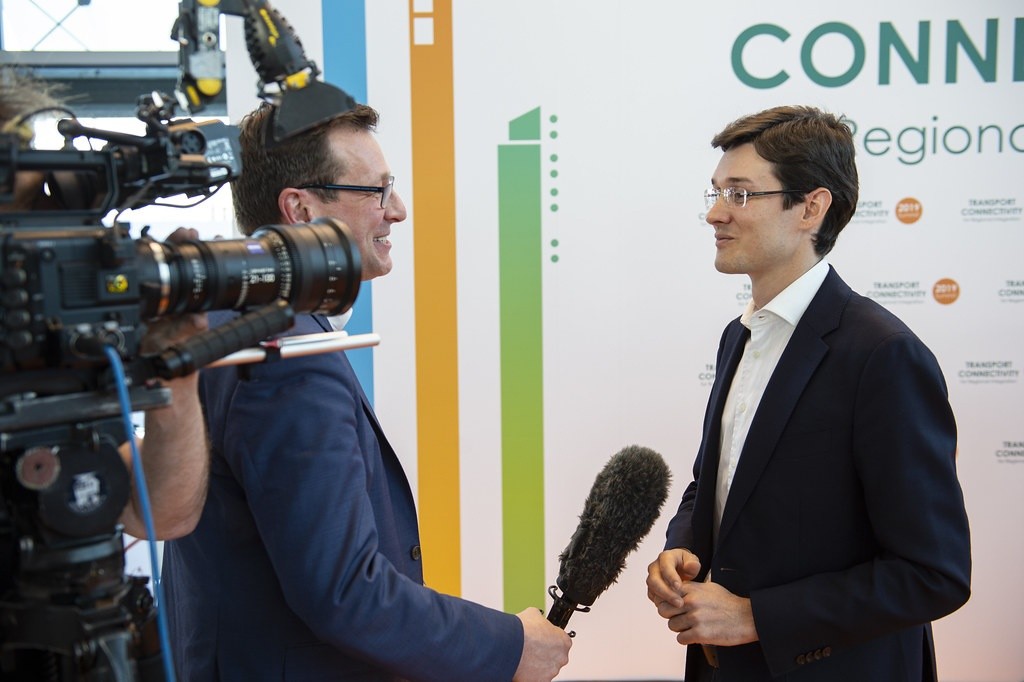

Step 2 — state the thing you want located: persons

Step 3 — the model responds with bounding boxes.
[0,81,222,541]
[157,93,572,682]
[646,106,972,682]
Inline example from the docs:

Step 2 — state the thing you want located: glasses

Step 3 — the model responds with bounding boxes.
[705,187,815,210]
[294,175,395,208]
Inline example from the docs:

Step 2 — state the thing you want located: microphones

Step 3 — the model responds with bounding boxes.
[552,446,671,607]
